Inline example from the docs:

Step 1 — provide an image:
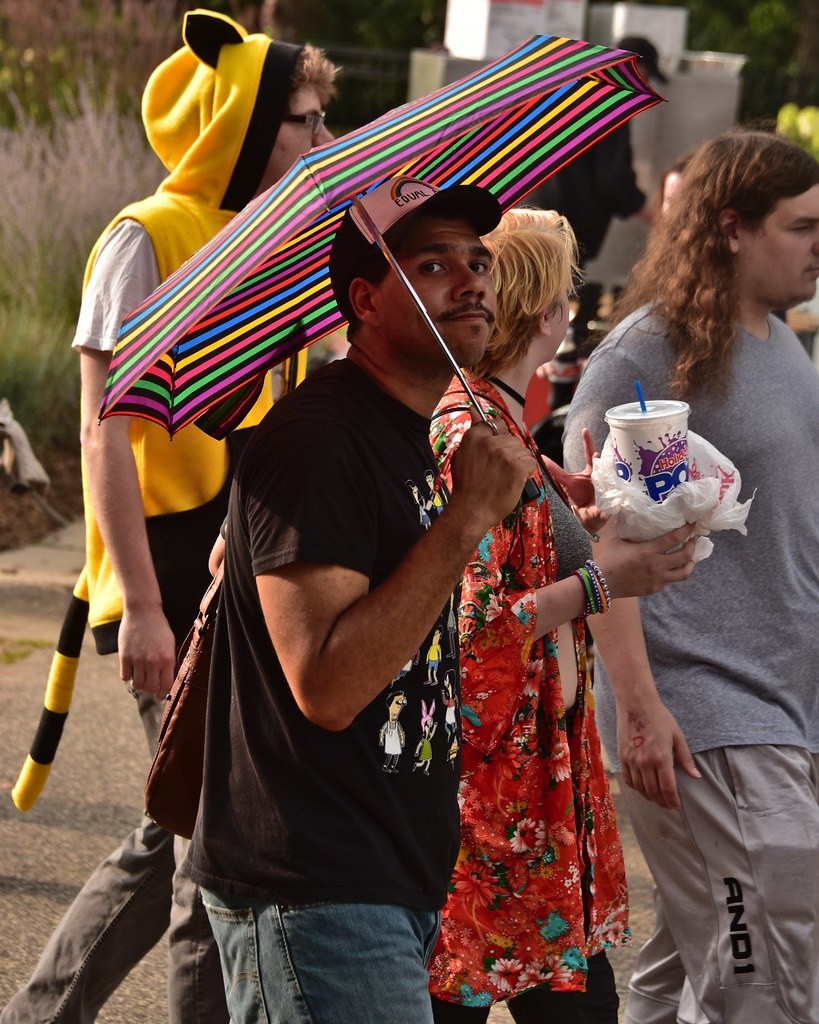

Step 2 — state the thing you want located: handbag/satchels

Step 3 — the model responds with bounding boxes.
[144,614,214,839]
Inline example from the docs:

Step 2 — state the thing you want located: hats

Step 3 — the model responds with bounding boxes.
[619,35,669,86]
[329,175,502,319]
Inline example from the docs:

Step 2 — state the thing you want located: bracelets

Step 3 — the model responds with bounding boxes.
[574,559,610,619]
[571,505,600,544]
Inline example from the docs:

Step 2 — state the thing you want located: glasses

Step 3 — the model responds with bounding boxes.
[565,274,586,298]
[285,109,326,136]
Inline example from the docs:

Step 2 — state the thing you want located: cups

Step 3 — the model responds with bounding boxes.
[605,400,690,501]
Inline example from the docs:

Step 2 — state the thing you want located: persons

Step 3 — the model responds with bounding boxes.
[565,132,819,1024]
[0,9,534,1024]
[428,210,701,1024]
[529,34,694,363]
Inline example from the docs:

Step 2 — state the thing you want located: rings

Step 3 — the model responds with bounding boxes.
[484,421,499,435]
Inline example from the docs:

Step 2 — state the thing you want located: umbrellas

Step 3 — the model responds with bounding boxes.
[97,31,668,511]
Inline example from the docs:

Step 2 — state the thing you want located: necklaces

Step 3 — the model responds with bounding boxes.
[484,372,526,408]
